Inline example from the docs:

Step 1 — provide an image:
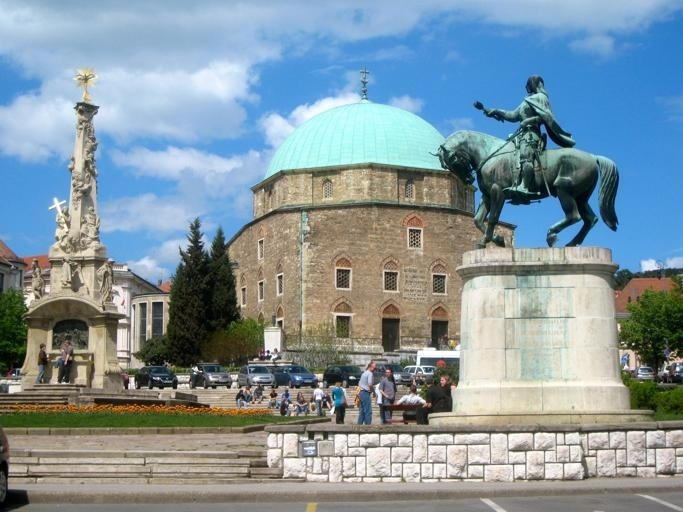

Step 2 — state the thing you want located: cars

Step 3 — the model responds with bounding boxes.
[270,363,444,390]
[120,366,130,390]
[232,363,277,390]
[635,359,683,382]
[132,365,181,392]
[0,424,13,505]
[187,361,233,390]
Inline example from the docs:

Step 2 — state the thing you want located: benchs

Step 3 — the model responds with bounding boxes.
[378,403,425,425]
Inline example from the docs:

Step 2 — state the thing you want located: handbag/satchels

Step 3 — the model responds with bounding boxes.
[340,394,350,408]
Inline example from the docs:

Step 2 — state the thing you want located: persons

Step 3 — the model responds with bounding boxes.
[192,365,203,389]
[484,76,576,194]
[121,372,129,390]
[35,343,48,384]
[31,122,114,302]
[61,340,73,384]
[235,347,456,424]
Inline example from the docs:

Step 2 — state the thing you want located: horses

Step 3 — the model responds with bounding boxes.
[429,129,619,250]
